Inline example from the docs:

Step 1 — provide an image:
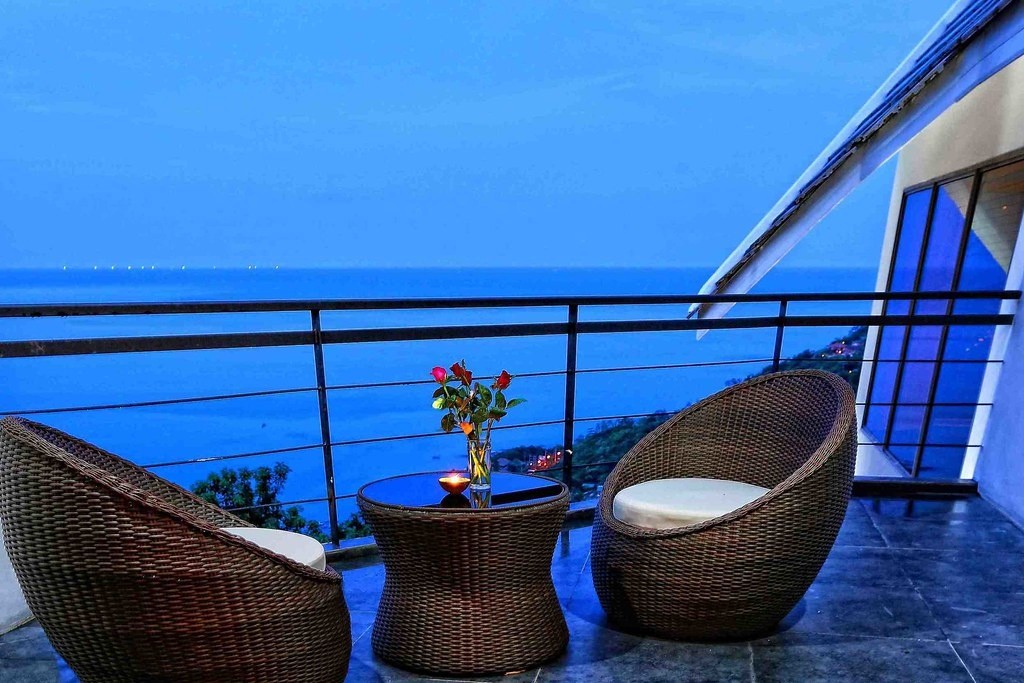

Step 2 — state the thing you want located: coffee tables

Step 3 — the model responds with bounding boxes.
[357,469,574,678]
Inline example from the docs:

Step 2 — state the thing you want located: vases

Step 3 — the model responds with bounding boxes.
[467,438,492,490]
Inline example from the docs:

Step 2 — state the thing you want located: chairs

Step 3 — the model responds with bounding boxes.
[589,369,860,642]
[0,414,352,682]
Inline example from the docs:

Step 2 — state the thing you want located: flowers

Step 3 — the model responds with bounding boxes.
[428,358,528,485]
